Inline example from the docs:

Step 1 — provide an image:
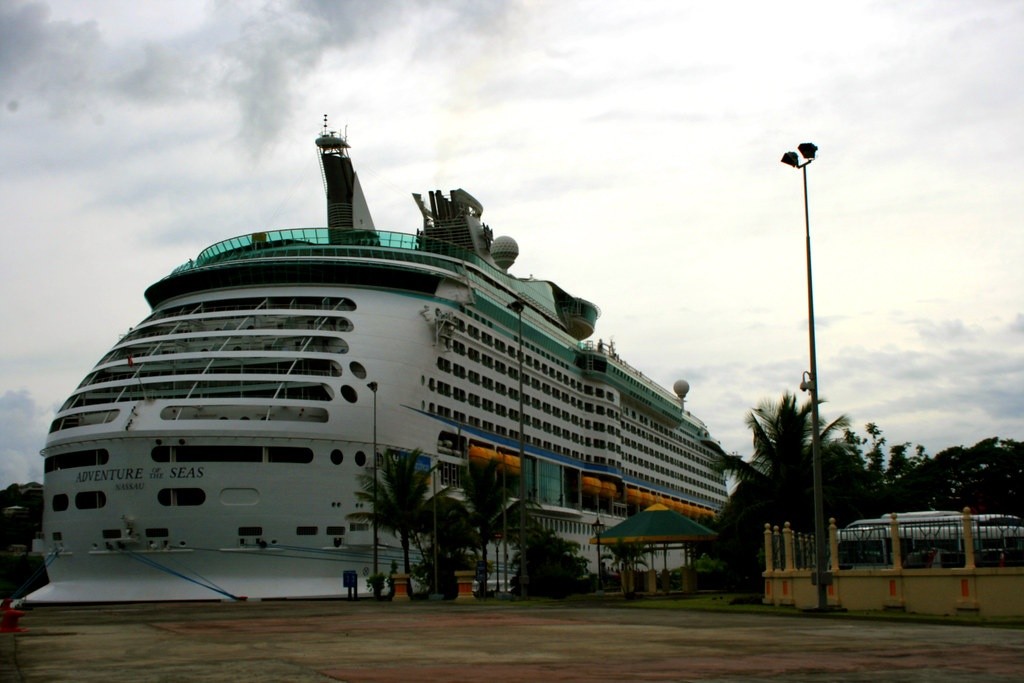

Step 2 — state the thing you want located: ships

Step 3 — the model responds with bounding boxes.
[4,109,732,610]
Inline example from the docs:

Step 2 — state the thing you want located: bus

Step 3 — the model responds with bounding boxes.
[836,508,1024,567]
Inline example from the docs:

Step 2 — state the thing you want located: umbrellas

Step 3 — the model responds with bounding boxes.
[590,503,719,593]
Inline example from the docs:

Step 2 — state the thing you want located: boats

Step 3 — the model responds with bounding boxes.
[628,488,643,505]
[581,476,603,493]
[469,444,505,472]
[497,452,521,475]
[642,492,716,521]
[601,481,617,498]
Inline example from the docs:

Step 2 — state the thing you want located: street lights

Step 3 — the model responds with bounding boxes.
[778,142,834,612]
[590,518,604,590]
[366,381,378,575]
[509,301,531,603]
